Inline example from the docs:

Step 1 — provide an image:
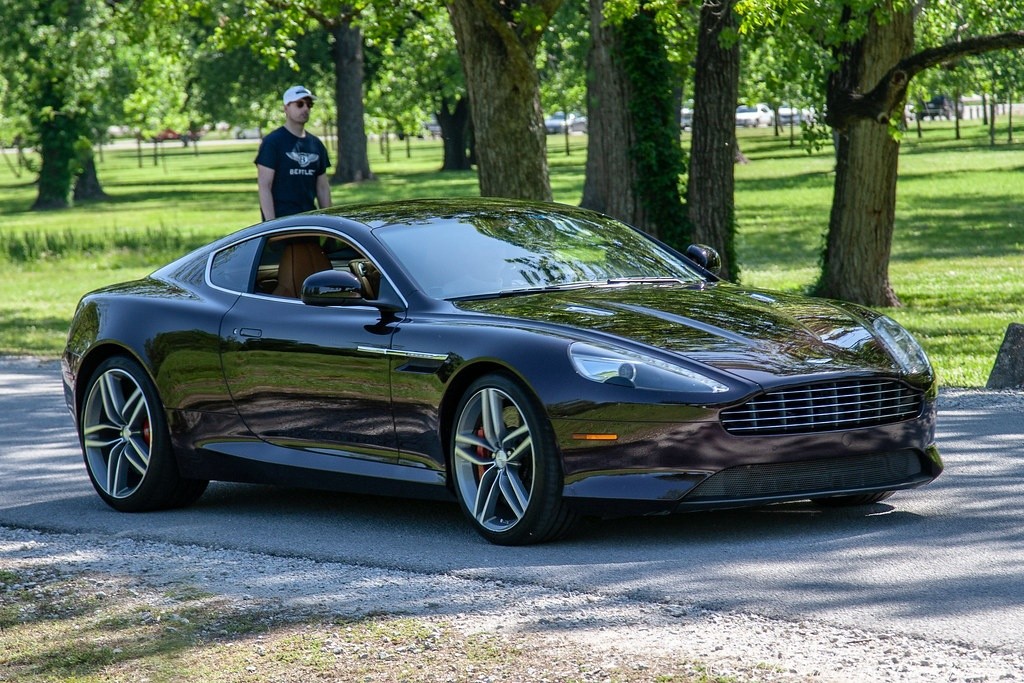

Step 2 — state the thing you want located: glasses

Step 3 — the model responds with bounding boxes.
[294,101,314,108]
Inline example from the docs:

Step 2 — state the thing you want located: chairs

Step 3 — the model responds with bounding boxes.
[271,246,333,299]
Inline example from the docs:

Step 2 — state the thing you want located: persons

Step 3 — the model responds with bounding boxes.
[253,86,332,222]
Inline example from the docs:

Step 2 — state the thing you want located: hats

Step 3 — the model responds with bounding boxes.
[283,86,316,105]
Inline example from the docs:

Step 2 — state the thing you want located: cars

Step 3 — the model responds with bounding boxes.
[733,103,812,126]
[547,109,589,134]
[61,195,943,547]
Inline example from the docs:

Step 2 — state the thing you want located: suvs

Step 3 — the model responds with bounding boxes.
[920,95,963,121]
